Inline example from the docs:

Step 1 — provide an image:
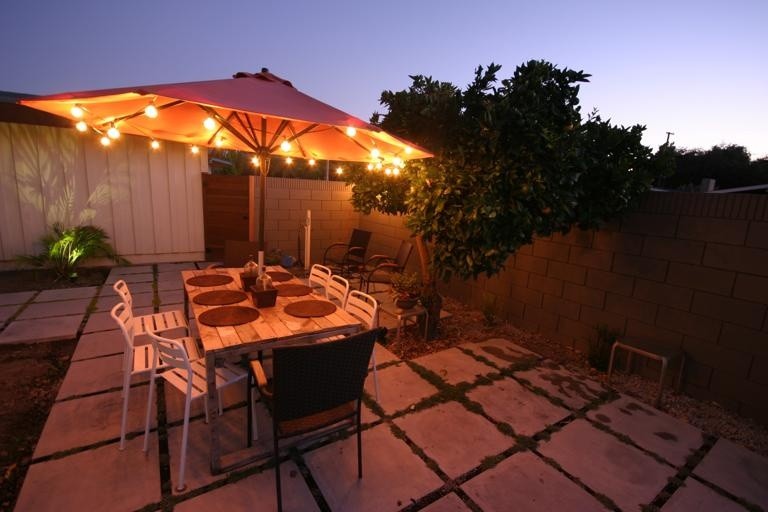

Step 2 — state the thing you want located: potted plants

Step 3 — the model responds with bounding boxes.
[387,271,424,314]
[588,322,621,372]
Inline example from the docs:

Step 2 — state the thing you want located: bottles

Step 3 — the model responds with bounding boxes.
[243,254,259,277]
[255,266,272,292]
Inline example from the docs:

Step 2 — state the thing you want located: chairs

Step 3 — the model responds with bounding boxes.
[322,227,414,302]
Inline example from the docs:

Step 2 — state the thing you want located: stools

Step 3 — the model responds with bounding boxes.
[376,301,429,349]
[607,336,686,410]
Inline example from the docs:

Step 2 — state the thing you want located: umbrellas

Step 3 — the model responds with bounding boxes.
[15,65,437,280]
